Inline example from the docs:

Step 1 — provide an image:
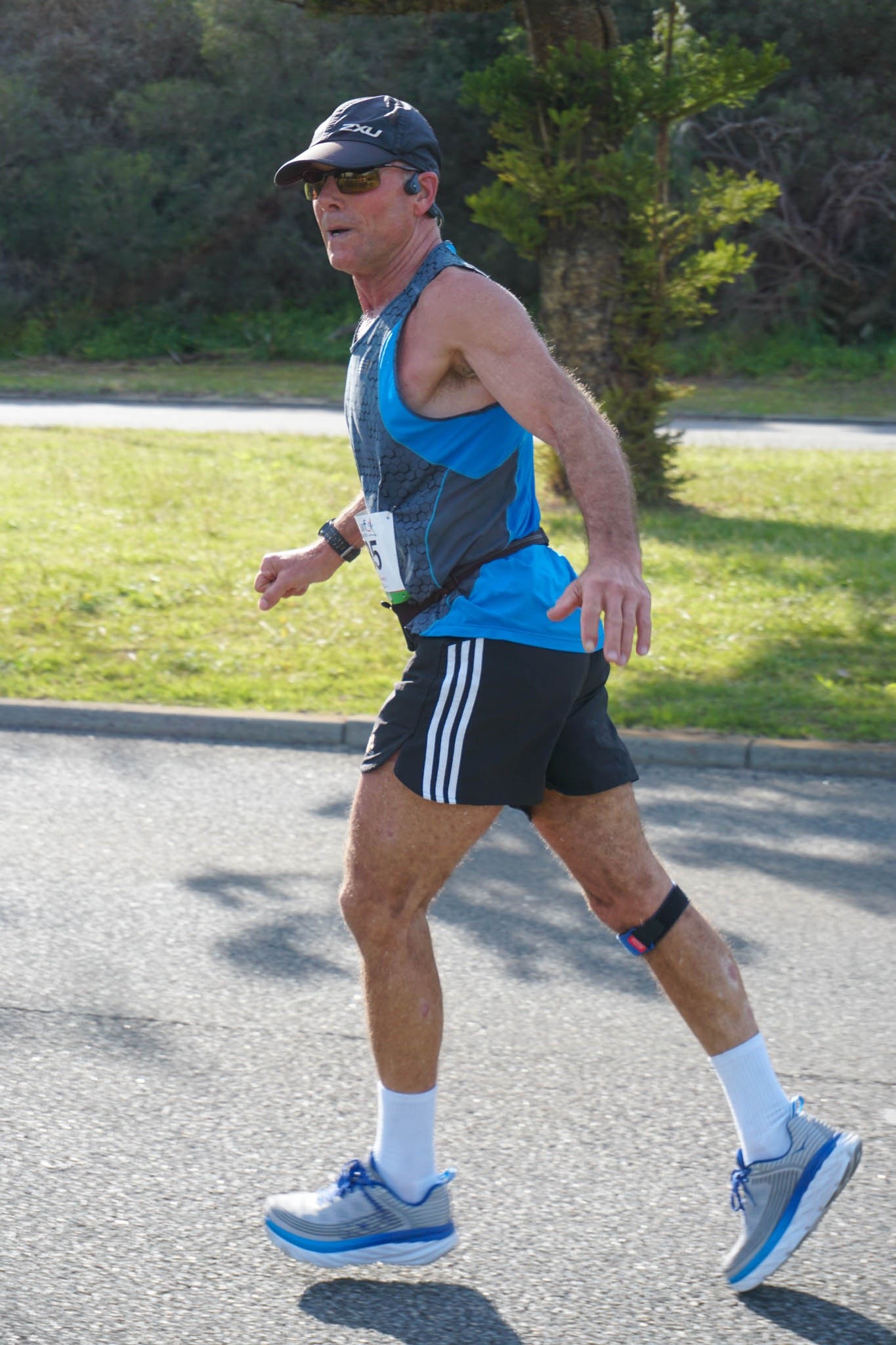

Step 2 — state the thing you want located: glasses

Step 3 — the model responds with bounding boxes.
[301,163,421,200]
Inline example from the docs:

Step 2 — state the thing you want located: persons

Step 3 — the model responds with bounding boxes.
[254,96,864,1298]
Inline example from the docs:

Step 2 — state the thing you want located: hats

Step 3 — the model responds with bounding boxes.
[274,94,441,187]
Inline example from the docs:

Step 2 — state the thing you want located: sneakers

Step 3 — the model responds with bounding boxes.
[261,1150,458,1267]
[718,1096,862,1295]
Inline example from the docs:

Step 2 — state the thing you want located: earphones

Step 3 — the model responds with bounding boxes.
[404,173,421,196]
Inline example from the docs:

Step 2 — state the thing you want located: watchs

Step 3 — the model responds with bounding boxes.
[318,517,361,564]
